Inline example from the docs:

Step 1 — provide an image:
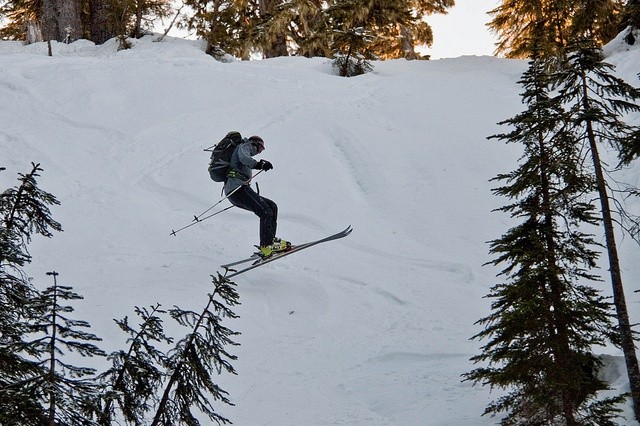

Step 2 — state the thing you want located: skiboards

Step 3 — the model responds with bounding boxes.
[221,225,353,283]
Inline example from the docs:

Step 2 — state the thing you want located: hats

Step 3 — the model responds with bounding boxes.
[248,136,265,149]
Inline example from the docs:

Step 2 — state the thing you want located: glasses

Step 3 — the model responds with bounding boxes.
[258,145,264,153]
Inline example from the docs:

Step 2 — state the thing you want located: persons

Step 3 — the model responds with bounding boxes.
[225,136,291,260]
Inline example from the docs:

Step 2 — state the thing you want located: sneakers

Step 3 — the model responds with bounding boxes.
[274,239,286,246]
[259,242,287,257]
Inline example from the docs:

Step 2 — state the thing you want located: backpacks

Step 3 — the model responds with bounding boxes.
[208,131,244,182]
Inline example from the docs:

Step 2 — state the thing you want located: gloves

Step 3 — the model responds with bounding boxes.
[255,159,273,171]
